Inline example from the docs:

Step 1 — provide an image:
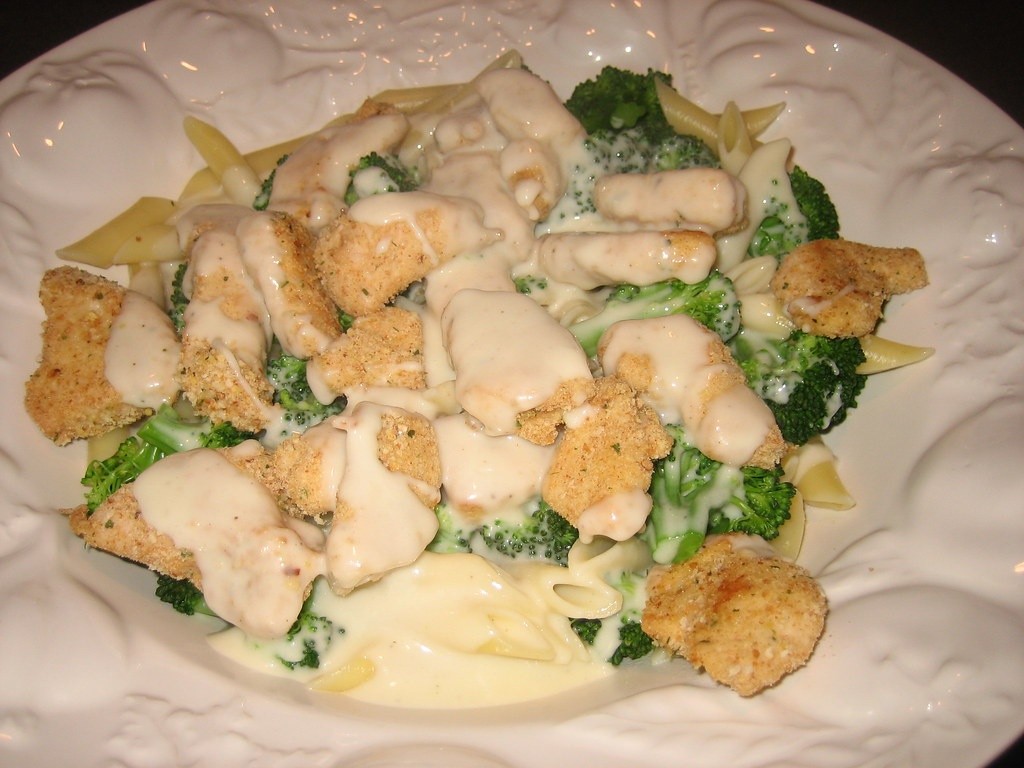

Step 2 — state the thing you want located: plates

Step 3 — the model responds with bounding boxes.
[0,0,1024,768]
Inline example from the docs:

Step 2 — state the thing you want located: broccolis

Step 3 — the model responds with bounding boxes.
[77,67,873,665]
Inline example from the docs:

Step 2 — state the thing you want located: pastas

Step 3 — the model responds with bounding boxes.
[53,77,934,560]
